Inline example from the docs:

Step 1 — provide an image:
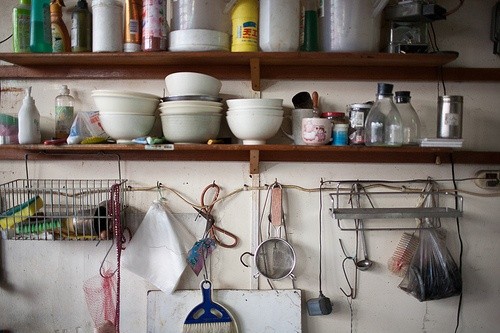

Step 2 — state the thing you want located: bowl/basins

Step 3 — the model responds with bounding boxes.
[90,71,284,143]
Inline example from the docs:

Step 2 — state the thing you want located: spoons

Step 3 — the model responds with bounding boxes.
[350,184,373,271]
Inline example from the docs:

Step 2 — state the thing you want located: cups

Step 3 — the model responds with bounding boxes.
[301,118,333,145]
[436,95,463,140]
[280,109,316,145]
[70,206,110,236]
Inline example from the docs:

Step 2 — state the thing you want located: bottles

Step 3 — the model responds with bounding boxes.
[333,123,349,146]
[53,85,75,142]
[320,111,348,140]
[363,82,403,148]
[10,0,390,51]
[394,91,421,145]
[18,86,42,145]
[347,103,375,146]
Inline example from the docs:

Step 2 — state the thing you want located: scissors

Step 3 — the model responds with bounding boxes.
[193,184,239,249]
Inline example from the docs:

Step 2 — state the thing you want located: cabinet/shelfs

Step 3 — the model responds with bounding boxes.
[0,49,500,165]
